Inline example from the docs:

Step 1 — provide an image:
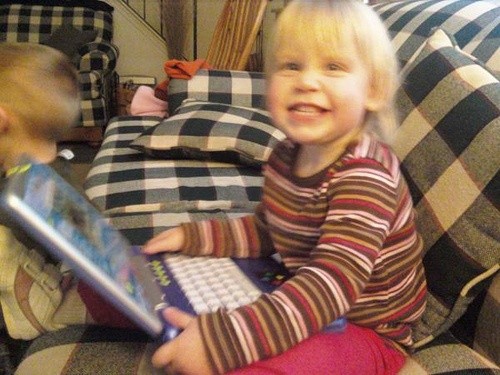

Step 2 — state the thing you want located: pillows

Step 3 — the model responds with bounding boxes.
[39,24,98,60]
[370,27,500,349]
[128,99,287,167]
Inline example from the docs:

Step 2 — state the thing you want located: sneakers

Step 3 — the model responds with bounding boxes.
[0,225,64,341]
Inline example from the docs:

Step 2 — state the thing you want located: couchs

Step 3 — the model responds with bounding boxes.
[0,0,120,147]
[13,0,500,375]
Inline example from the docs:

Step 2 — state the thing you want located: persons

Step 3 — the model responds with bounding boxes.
[0,40,101,265]
[1,0,430,375]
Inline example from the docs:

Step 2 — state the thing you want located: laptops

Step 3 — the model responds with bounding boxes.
[1,153,346,343]
